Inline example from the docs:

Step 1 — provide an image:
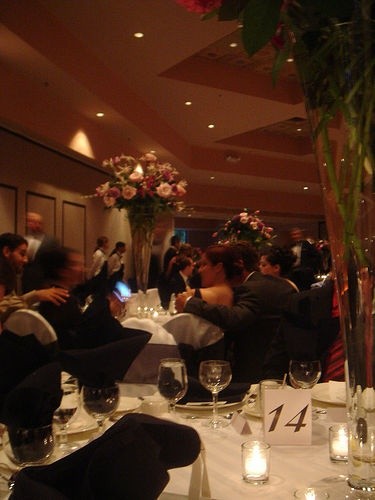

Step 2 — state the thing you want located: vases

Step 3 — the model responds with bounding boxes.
[291,25,375,500]
[126,211,159,312]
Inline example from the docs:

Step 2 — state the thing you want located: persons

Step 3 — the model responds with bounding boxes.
[0,232,124,348]
[88,235,109,294]
[164,235,181,271]
[108,241,126,293]
[287,227,314,289]
[166,241,331,380]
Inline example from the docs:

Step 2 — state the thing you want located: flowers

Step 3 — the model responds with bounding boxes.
[96,149,189,294]
[212,207,277,251]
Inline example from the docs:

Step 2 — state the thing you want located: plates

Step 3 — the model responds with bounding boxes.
[53,412,99,435]
[175,395,247,410]
[311,384,348,406]
[116,396,143,413]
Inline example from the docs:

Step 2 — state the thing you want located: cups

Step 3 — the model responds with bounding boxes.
[293,488,330,500]
[2,424,55,467]
[329,425,349,464]
[135,305,163,322]
[259,379,286,412]
[241,441,272,485]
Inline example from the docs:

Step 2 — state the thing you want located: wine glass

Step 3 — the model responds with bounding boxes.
[199,360,232,428]
[289,359,322,420]
[157,358,188,423]
[51,377,81,452]
[81,382,119,432]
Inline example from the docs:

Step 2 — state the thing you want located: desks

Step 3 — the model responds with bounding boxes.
[114,309,182,385]
[0,380,375,500]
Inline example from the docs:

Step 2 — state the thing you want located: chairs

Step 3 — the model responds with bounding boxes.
[0,276,335,399]
